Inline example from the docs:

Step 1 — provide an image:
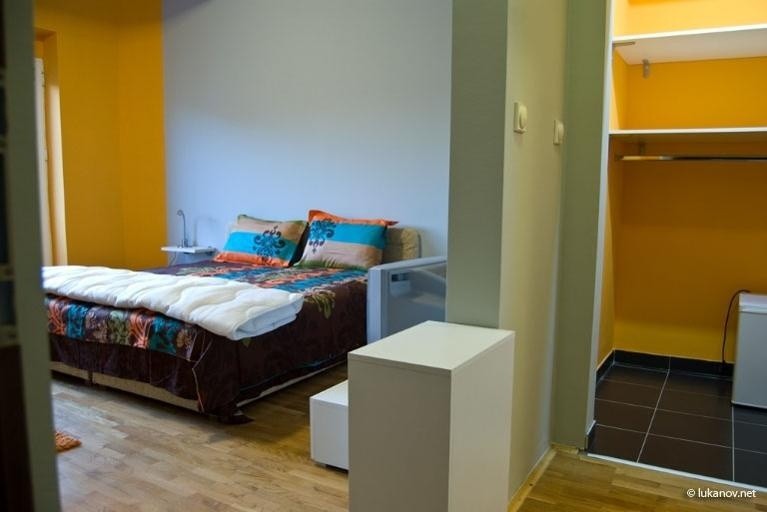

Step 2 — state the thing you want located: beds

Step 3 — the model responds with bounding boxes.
[42,227,421,427]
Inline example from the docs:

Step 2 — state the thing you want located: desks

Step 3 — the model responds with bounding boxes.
[160,245,216,266]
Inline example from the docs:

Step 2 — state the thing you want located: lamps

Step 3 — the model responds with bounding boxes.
[177,210,188,247]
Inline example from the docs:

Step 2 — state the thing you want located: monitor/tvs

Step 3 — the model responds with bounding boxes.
[368,255,446,344]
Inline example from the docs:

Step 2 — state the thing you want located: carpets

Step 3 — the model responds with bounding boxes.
[54,431,81,451]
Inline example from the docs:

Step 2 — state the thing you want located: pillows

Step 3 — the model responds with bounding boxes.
[212,214,308,268]
[293,210,399,270]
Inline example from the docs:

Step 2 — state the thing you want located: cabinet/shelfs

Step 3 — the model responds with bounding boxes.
[348,320,519,512]
[729,292,766,411]
[308,380,347,470]
[609,23,766,162]
